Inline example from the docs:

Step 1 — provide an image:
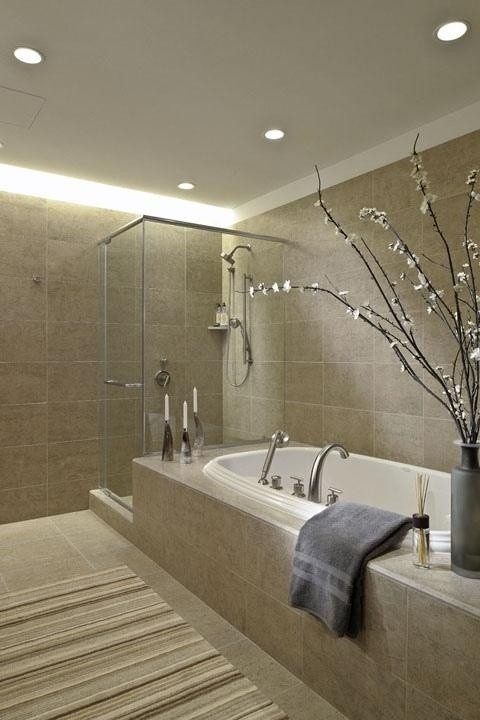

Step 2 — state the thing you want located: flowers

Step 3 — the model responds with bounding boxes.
[236,133,480,443]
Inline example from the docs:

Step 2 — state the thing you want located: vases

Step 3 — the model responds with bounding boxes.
[450,440,480,580]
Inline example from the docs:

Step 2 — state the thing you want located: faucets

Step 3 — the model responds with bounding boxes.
[307,442,350,504]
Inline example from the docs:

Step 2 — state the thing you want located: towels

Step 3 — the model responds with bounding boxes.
[286,500,412,638]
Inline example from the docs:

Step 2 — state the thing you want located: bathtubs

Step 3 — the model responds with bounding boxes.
[202,444,453,553]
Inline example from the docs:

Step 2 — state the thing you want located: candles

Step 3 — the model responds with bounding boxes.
[164,386,198,433]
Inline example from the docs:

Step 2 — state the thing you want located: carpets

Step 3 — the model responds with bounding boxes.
[0,566,288,720]
